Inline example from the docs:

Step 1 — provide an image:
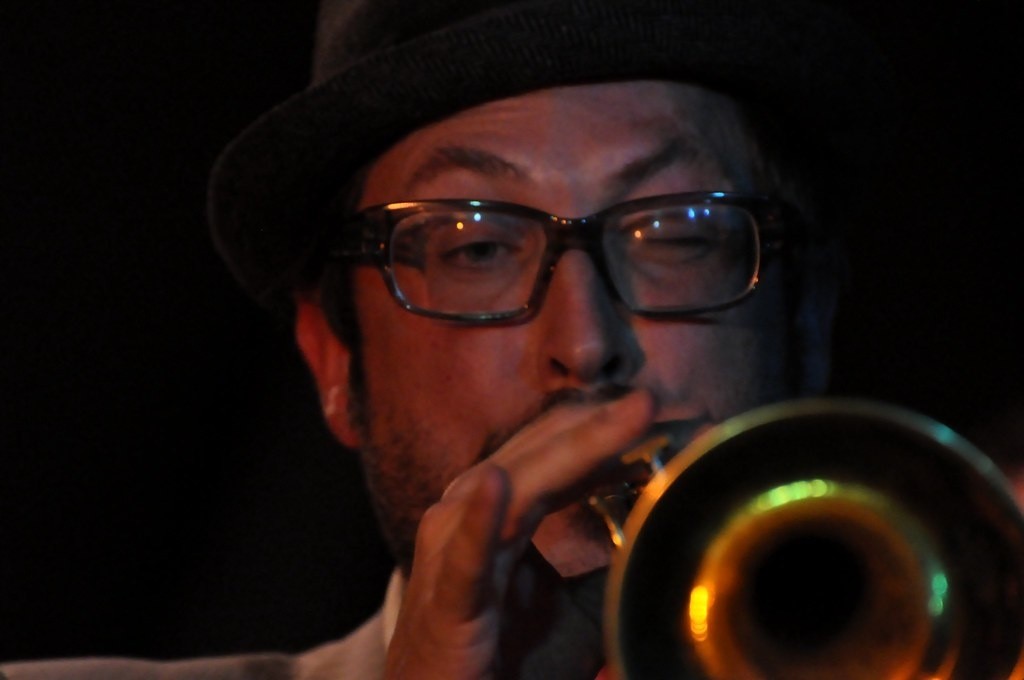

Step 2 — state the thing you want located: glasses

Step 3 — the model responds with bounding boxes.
[331,190,816,320]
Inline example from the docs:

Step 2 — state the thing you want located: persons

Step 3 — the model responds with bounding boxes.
[0,0,1024,680]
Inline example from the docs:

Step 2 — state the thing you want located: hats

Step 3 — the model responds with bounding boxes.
[204,0,901,297]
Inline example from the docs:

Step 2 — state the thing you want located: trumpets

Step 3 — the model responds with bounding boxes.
[575,394,1024,680]
